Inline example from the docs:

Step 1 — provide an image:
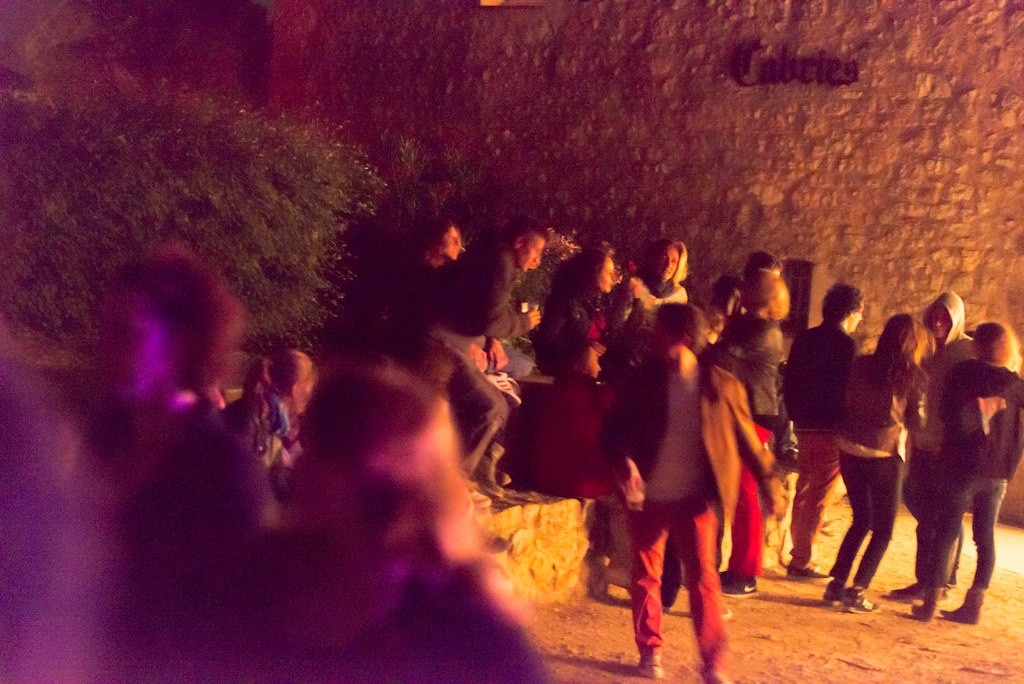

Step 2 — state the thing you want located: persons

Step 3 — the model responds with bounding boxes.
[890,290,980,601]
[462,216,789,601]
[784,283,864,579]
[222,351,315,470]
[824,314,925,613]
[913,322,1024,624]
[75,242,275,682]
[202,366,554,684]
[390,220,461,366]
[602,303,787,684]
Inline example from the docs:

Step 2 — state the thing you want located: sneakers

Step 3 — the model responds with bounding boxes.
[787,564,833,584]
[721,579,758,598]
[824,582,849,605]
[719,571,738,585]
[843,588,881,613]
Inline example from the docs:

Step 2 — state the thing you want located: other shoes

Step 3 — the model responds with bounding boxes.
[637,660,663,678]
[472,465,506,498]
[891,582,929,600]
[912,604,933,622]
[761,564,788,581]
[599,563,633,587]
[583,549,608,575]
[464,482,491,508]
[494,467,512,487]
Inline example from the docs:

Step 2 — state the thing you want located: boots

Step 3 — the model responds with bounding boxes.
[939,588,982,624]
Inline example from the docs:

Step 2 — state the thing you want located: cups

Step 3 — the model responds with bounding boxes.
[528,303,539,311]
